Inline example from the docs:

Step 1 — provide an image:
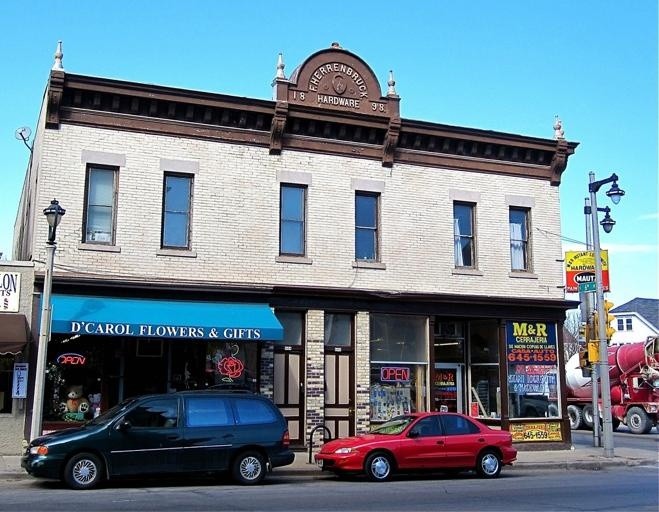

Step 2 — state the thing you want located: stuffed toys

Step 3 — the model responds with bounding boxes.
[58,384,90,414]
[89,392,102,411]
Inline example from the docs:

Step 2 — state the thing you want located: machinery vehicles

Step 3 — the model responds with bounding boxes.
[546,334,659,435]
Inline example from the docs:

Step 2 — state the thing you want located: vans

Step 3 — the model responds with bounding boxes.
[21,390,295,490]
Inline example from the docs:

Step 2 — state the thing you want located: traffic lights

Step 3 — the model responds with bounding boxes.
[578,347,590,369]
[594,312,599,339]
[603,299,616,342]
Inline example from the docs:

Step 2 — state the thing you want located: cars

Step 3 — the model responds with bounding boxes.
[314,411,518,481]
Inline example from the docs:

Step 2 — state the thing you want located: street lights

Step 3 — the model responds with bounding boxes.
[589,171,625,458]
[28,197,67,445]
[585,196,616,447]
